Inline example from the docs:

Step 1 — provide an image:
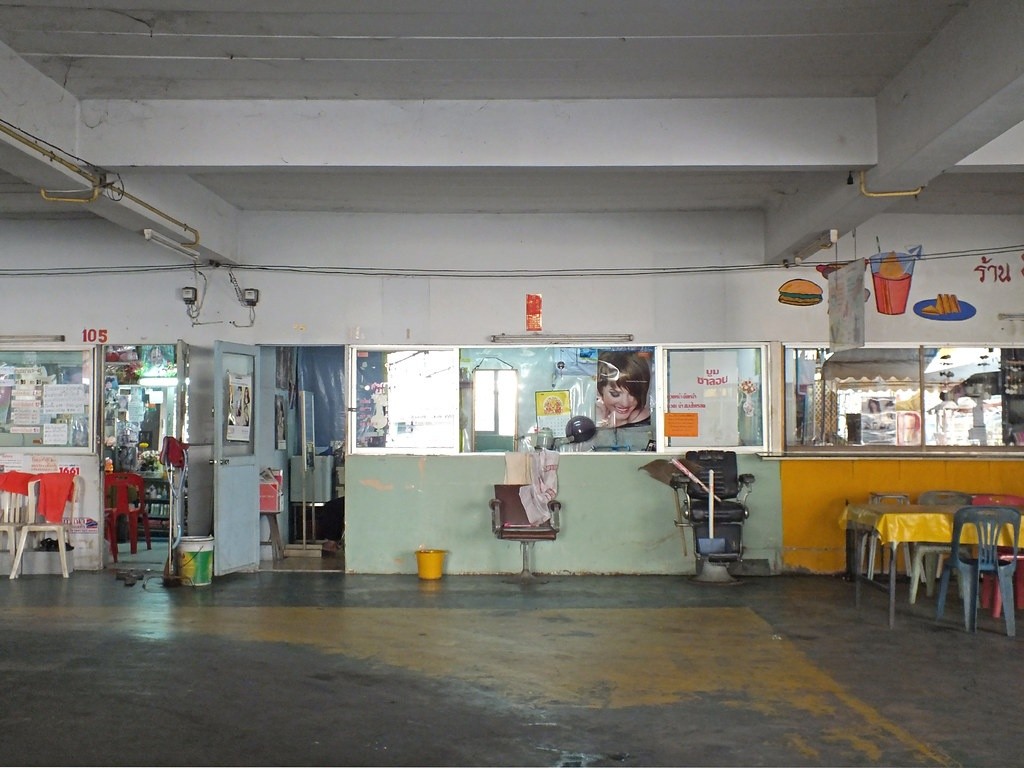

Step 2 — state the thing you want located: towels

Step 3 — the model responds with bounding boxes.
[503,448,562,528]
[1,469,79,525]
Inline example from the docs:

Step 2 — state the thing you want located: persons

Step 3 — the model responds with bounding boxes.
[277,402,287,439]
[145,346,169,376]
[230,386,251,425]
[597,352,651,428]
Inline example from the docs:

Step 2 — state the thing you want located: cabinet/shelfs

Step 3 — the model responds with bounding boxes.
[118,476,188,536]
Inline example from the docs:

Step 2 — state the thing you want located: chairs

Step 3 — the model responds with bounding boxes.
[674,450,756,587]
[104,471,151,555]
[970,493,1024,618]
[488,484,562,585]
[0,473,37,579]
[907,493,984,606]
[8,475,79,580]
[934,506,1020,635]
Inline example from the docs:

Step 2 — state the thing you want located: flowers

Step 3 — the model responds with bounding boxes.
[115,364,143,380]
[137,443,159,473]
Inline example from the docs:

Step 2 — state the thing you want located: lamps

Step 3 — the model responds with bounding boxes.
[143,229,200,260]
[0,335,65,343]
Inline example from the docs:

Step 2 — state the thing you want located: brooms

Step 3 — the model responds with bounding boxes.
[637,458,704,557]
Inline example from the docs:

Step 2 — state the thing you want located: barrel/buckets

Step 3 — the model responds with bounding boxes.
[415,549,449,580]
[174,536,215,586]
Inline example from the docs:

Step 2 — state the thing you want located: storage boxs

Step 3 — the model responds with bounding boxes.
[290,454,335,501]
[260,468,284,512]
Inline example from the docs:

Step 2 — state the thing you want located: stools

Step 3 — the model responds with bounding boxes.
[260,511,283,560]
[865,491,915,580]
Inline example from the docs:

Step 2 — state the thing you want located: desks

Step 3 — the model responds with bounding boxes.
[839,504,1024,627]
[293,502,326,544]
